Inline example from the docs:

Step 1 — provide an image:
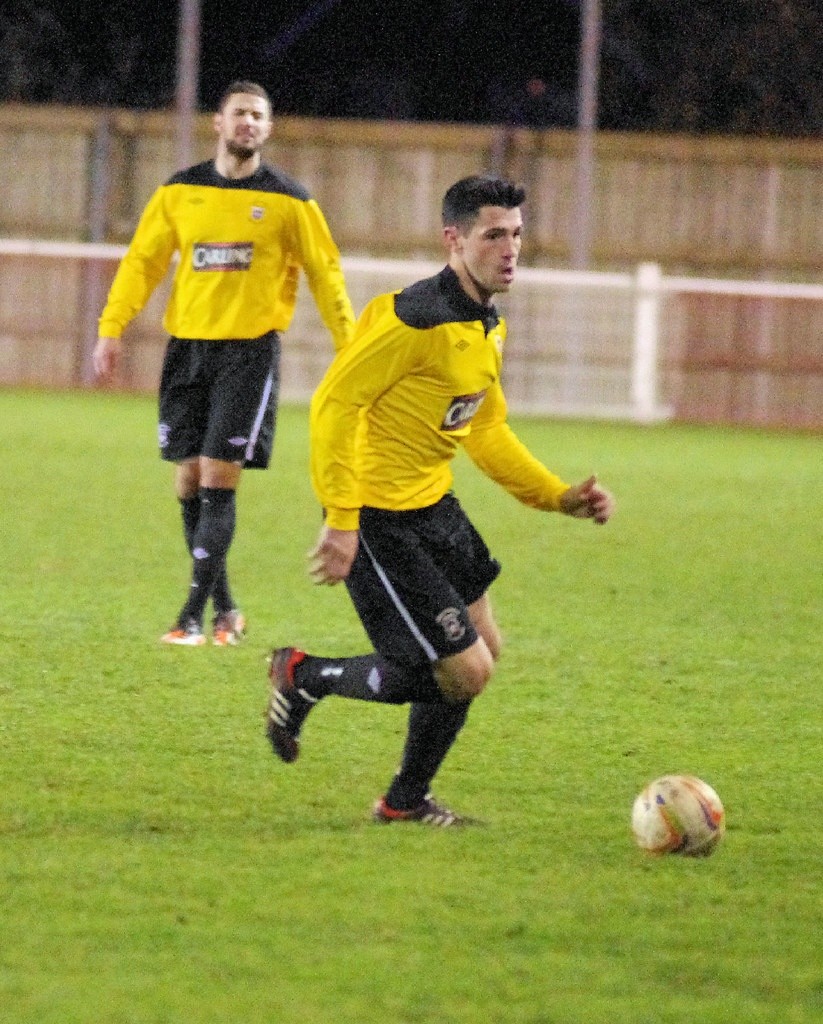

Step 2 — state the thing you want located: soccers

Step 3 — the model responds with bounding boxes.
[631,773,726,860]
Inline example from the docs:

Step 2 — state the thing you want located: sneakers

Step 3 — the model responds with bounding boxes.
[266,645,319,764]
[210,609,245,645]
[161,619,207,647]
[376,790,477,828]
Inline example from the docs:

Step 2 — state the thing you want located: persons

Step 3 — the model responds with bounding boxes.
[265,175,613,831]
[92,81,356,644]
